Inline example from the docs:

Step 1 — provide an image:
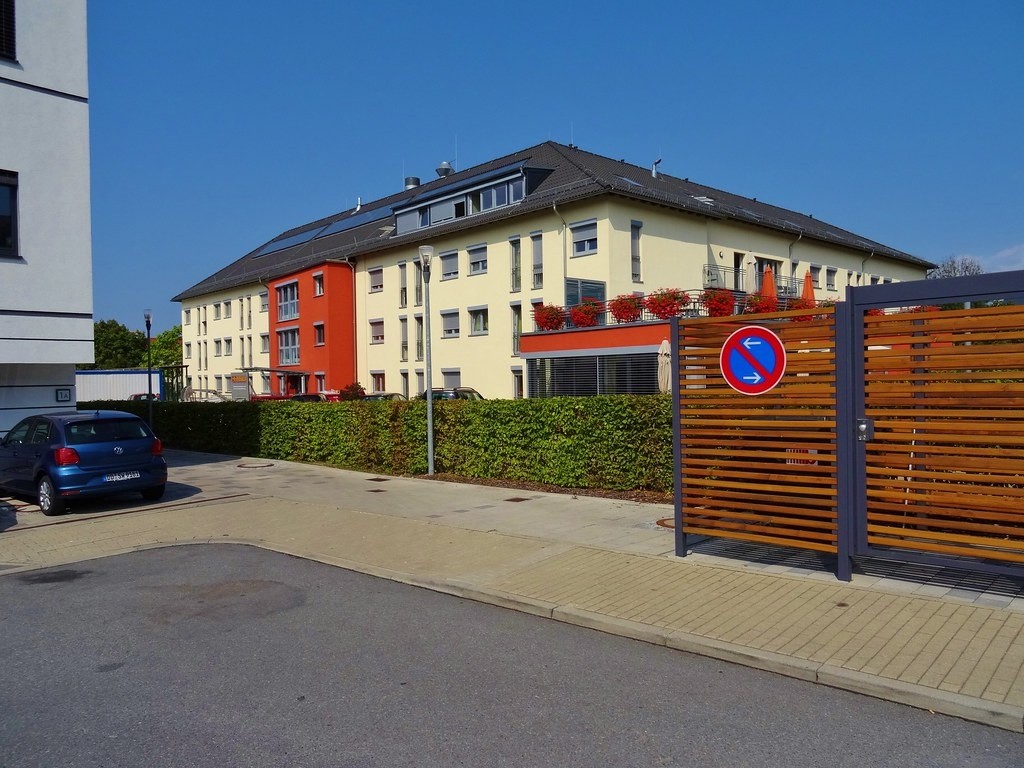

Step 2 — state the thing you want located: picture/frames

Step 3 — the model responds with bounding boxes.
[56,389,71,402]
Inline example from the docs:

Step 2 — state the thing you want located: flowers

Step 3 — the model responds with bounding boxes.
[608,294,647,325]
[740,290,778,314]
[647,288,693,320]
[570,296,607,328]
[786,295,817,323]
[698,288,736,318]
[534,301,566,333]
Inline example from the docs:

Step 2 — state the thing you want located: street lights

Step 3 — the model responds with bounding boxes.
[419,245,434,477]
[144,309,153,431]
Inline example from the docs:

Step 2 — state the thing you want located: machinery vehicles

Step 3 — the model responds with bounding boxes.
[178,365,305,400]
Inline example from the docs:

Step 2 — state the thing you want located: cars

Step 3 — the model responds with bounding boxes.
[0,410,167,515]
[362,393,409,401]
[128,394,156,400]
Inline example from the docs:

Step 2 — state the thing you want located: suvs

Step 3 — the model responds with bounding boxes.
[423,387,484,400]
[291,393,348,402]
